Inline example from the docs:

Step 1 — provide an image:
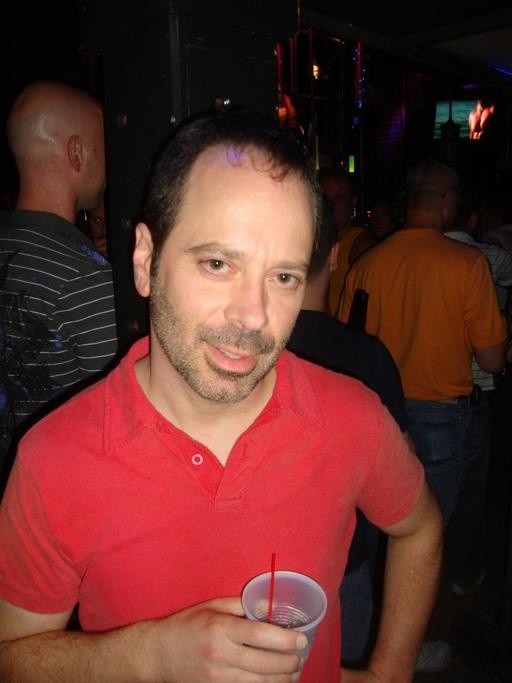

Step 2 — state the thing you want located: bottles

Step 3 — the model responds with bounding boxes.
[352,207,359,222]
[364,210,372,229]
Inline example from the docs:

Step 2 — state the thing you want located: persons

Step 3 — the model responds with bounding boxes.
[1,82,511,682]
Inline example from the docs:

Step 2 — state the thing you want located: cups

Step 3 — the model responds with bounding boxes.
[239,569,328,683]
